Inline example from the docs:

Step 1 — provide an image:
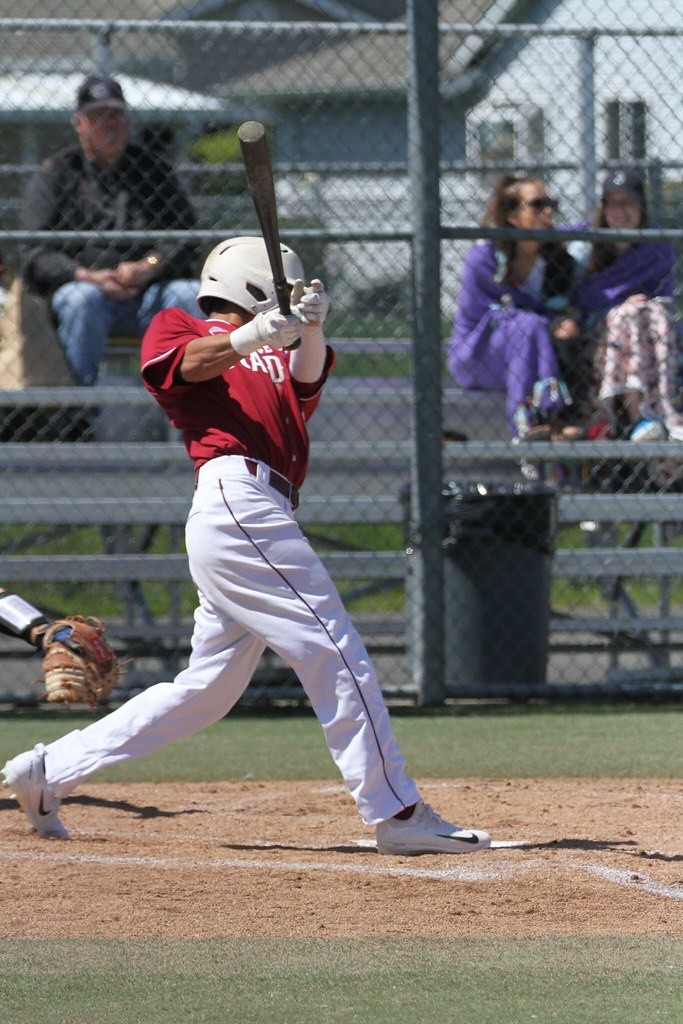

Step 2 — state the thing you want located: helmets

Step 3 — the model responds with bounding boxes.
[196,236,305,316]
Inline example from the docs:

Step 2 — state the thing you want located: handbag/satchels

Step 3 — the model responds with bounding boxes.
[0,243,75,390]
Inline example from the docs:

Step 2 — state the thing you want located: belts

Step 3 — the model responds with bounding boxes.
[244,459,299,512]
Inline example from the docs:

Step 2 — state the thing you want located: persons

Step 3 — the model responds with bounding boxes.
[1,236,491,857]
[445,168,593,438]
[580,169,683,448]
[12,72,209,384]
[0,583,119,705]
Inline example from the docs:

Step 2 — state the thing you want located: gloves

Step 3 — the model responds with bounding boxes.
[229,305,310,357]
[289,278,329,383]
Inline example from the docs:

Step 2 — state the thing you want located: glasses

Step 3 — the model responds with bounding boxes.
[526,198,558,210]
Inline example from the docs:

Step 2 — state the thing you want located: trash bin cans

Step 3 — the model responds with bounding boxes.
[398,477,559,686]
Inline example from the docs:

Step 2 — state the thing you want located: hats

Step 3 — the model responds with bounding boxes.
[79,75,126,113]
[601,169,643,203]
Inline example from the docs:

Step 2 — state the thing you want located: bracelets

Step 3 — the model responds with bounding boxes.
[144,254,161,274]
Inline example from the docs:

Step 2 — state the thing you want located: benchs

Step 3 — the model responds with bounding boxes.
[0,336,683,688]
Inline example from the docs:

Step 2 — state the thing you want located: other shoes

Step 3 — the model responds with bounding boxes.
[553,421,583,440]
[631,418,683,442]
[524,424,552,465]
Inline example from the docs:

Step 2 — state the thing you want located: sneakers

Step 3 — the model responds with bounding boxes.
[1,743,68,840]
[375,799,491,855]
